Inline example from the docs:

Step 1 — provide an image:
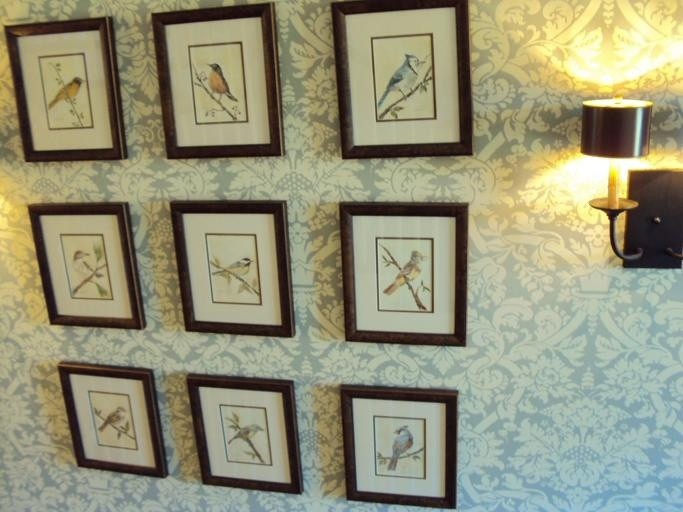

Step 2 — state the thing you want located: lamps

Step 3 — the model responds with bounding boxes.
[580,97,683,268]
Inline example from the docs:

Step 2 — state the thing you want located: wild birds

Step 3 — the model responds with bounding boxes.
[387,425,414,471]
[73,251,104,281]
[212,257,254,278]
[206,63,239,102]
[384,250,427,295]
[99,406,126,431]
[228,424,264,445]
[378,53,427,106]
[48,77,86,109]
